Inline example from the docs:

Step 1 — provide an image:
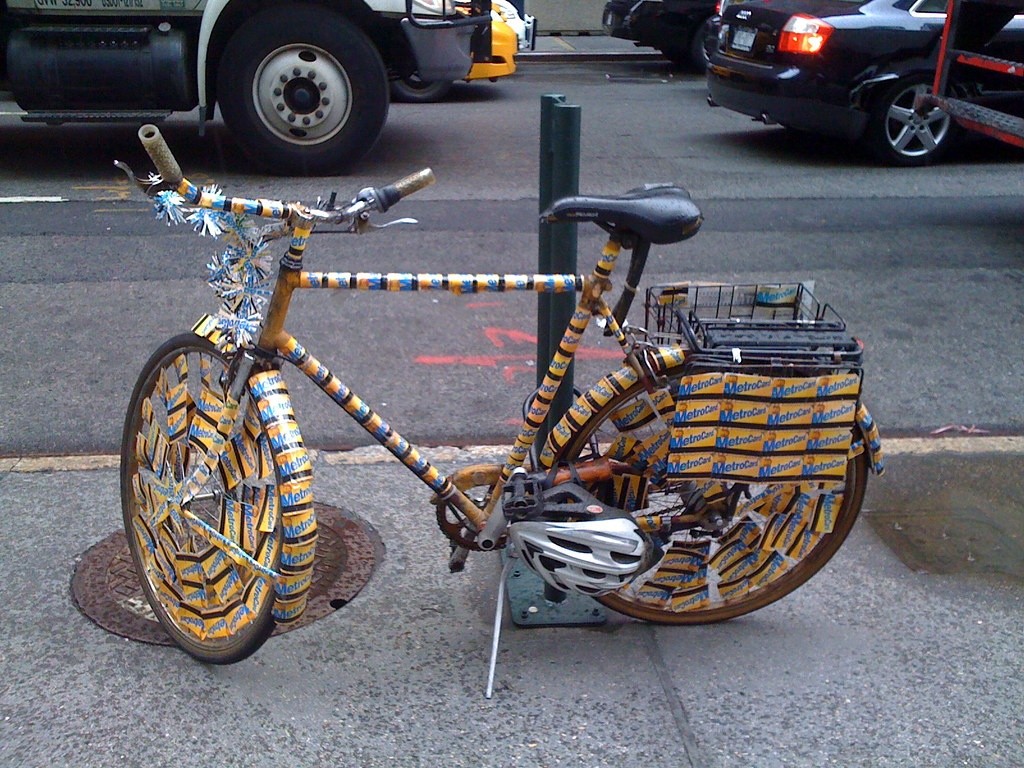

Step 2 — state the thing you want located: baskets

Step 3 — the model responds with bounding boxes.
[646,279,839,354]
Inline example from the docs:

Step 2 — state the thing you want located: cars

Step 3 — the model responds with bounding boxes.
[601,0,705,74]
[701,0,1024,166]
[388,0,538,105]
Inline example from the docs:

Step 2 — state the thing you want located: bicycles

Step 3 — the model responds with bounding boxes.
[110,123,884,667]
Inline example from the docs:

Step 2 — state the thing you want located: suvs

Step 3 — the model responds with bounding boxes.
[0,0,495,179]
